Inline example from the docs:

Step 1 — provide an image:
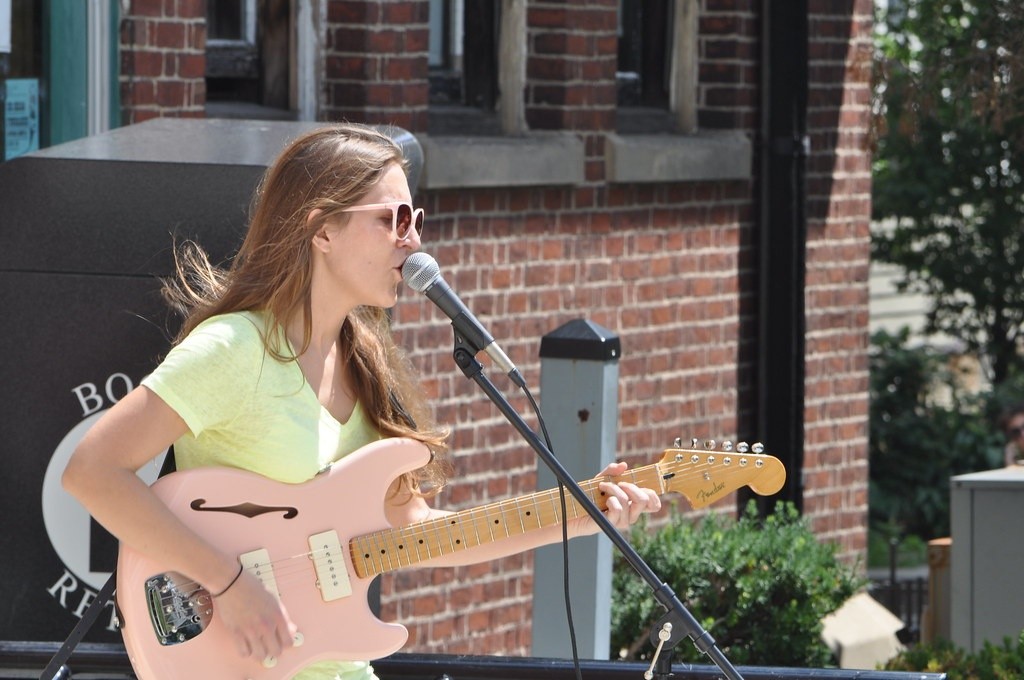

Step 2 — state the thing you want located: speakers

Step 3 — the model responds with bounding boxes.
[0,120,429,645]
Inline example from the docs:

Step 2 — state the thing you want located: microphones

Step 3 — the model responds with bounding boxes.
[401,251,526,388]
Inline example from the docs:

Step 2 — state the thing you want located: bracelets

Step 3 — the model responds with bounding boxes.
[212,566,243,599]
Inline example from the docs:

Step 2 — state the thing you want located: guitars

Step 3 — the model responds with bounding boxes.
[113,437,787,680]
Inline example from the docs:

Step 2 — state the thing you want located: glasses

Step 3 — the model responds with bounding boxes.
[344,201,425,242]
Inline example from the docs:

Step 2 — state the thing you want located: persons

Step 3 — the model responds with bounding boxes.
[61,125,661,680]
